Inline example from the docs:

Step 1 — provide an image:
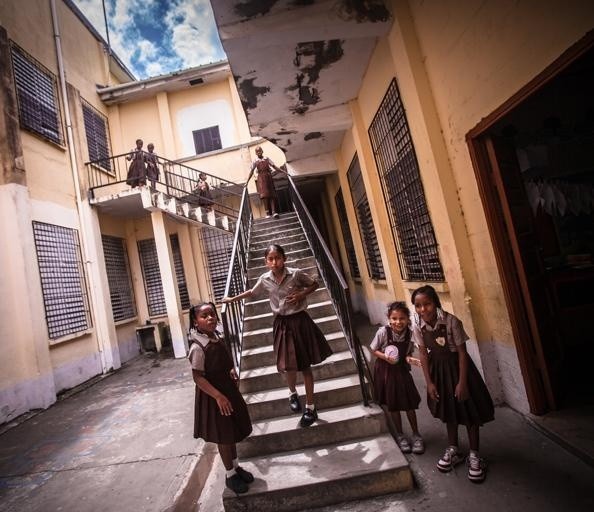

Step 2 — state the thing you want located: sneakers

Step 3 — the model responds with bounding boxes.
[265,214,271,219]
[272,213,279,218]
[466,453,486,481]
[435,447,464,472]
[412,434,424,453]
[397,434,411,452]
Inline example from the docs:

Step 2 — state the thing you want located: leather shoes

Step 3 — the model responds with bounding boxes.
[288,394,301,413]
[300,406,317,426]
[224,475,249,493]
[235,466,253,485]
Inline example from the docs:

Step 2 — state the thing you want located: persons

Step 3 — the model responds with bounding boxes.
[409,283,497,484]
[240,144,292,218]
[143,141,163,188]
[185,301,256,495]
[217,242,333,430]
[367,300,427,456]
[194,171,214,210]
[125,139,154,187]
